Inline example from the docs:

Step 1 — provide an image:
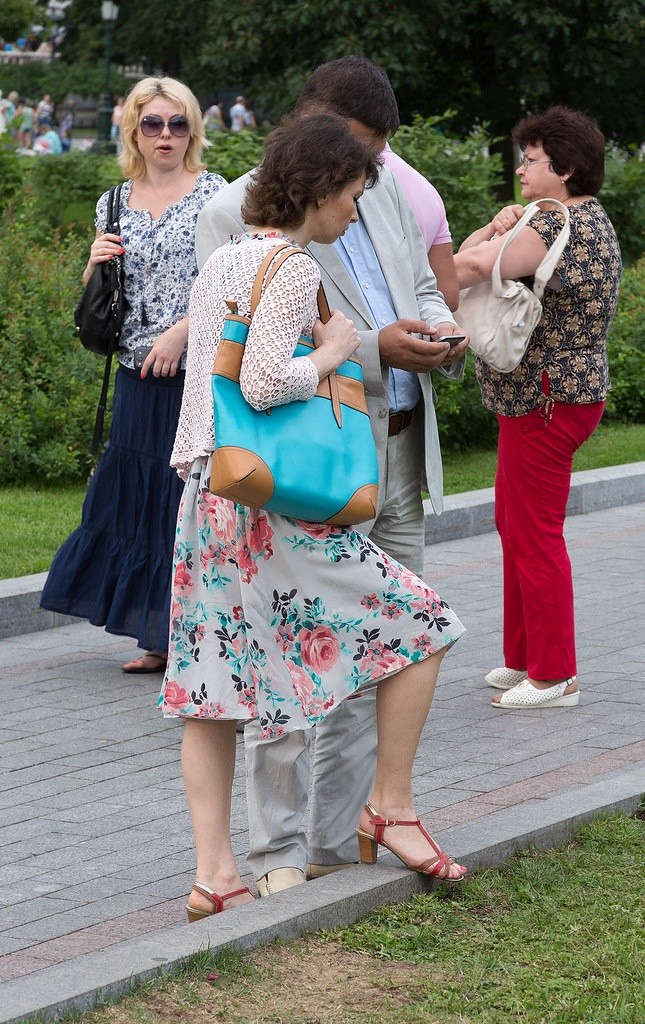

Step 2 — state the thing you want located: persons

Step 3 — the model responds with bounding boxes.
[157,107,468,921]
[229,95,257,132]
[195,54,466,896]
[0,90,75,154]
[203,100,226,131]
[41,72,229,674]
[376,141,459,310]
[111,95,126,140]
[454,107,622,709]
[0,32,54,55]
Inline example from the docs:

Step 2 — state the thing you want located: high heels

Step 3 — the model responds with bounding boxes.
[355,802,465,883]
[185,883,257,926]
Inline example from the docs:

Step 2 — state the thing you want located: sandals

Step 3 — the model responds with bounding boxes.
[122,646,167,675]
[482,667,532,688]
[490,676,579,709]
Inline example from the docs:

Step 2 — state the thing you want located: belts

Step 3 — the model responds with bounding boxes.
[386,404,417,437]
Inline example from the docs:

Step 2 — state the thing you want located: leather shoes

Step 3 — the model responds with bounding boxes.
[308,858,356,884]
[255,866,307,899]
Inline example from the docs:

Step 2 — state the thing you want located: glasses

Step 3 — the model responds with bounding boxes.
[137,114,192,138]
[520,154,554,169]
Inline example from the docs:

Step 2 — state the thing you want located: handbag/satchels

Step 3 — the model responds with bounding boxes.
[74,182,125,356]
[209,243,380,526]
[448,197,570,375]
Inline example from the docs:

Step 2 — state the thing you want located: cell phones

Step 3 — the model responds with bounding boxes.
[434,334,467,347]
[133,346,182,373]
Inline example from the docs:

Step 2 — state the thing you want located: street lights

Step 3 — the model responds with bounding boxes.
[91,0,120,156]
